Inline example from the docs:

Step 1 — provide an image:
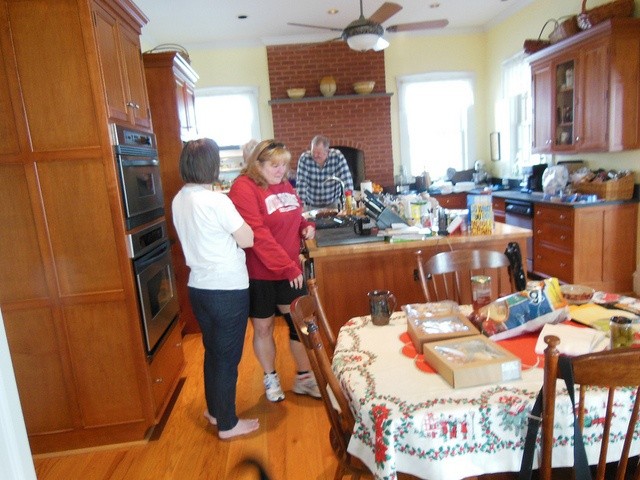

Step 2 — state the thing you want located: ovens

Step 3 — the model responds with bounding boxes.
[126,219,181,358]
[109,122,166,229]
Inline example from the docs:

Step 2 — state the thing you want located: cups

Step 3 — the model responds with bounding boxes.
[367,289,397,325]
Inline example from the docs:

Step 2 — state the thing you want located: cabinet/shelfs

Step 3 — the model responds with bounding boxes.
[526,16,639,154]
[216,146,248,192]
[531,199,639,294]
[1,0,185,454]
[141,50,199,331]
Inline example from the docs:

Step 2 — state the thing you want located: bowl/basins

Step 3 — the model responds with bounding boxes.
[353,80,376,96]
[559,284,595,305]
[286,88,306,98]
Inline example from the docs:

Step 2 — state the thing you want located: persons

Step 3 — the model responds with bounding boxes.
[227,139,323,402]
[171,137,260,439]
[241,138,259,164]
[295,135,354,213]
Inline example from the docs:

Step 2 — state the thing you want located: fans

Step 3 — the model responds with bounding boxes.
[287,1,448,54]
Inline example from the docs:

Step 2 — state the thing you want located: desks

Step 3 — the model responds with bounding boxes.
[302,197,533,361]
[327,284,640,480]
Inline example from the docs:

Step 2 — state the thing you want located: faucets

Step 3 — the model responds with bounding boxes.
[321,177,346,218]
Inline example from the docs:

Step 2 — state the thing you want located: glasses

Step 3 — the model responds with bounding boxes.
[257,143,284,160]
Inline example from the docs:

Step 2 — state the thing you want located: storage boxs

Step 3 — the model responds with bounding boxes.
[423,334,522,388]
[406,311,480,354]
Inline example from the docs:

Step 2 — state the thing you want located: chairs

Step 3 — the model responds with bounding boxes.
[414,242,526,307]
[290,294,377,480]
[540,336,640,480]
[299,252,336,350]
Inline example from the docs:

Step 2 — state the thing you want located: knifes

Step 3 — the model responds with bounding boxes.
[360,189,408,229]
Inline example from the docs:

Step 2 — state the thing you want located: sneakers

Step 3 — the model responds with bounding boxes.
[264,373,285,401]
[292,373,324,397]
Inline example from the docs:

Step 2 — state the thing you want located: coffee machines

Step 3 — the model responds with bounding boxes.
[522,164,547,193]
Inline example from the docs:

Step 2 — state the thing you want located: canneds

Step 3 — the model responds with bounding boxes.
[611,316,632,349]
